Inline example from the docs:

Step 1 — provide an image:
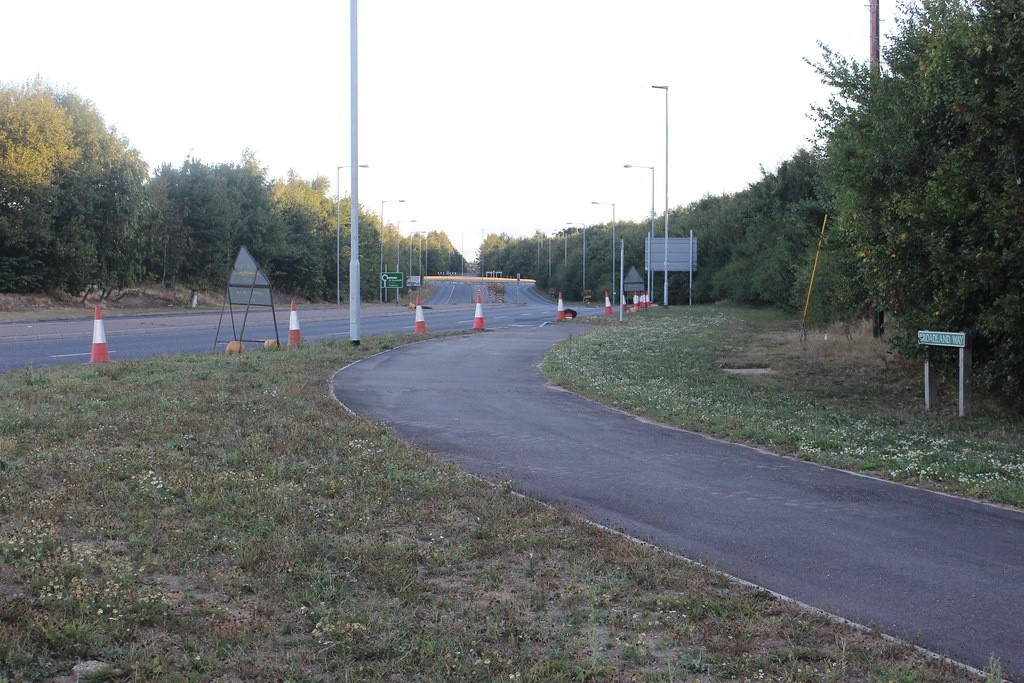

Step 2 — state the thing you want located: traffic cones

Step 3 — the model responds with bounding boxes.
[619,290,652,312]
[287,296,303,349]
[89,303,113,365]
[414,291,428,337]
[554,288,565,326]
[471,293,486,332]
[604,289,614,316]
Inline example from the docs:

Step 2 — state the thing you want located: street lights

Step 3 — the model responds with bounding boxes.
[378,193,406,305]
[651,81,672,311]
[533,159,657,303]
[335,162,365,307]
